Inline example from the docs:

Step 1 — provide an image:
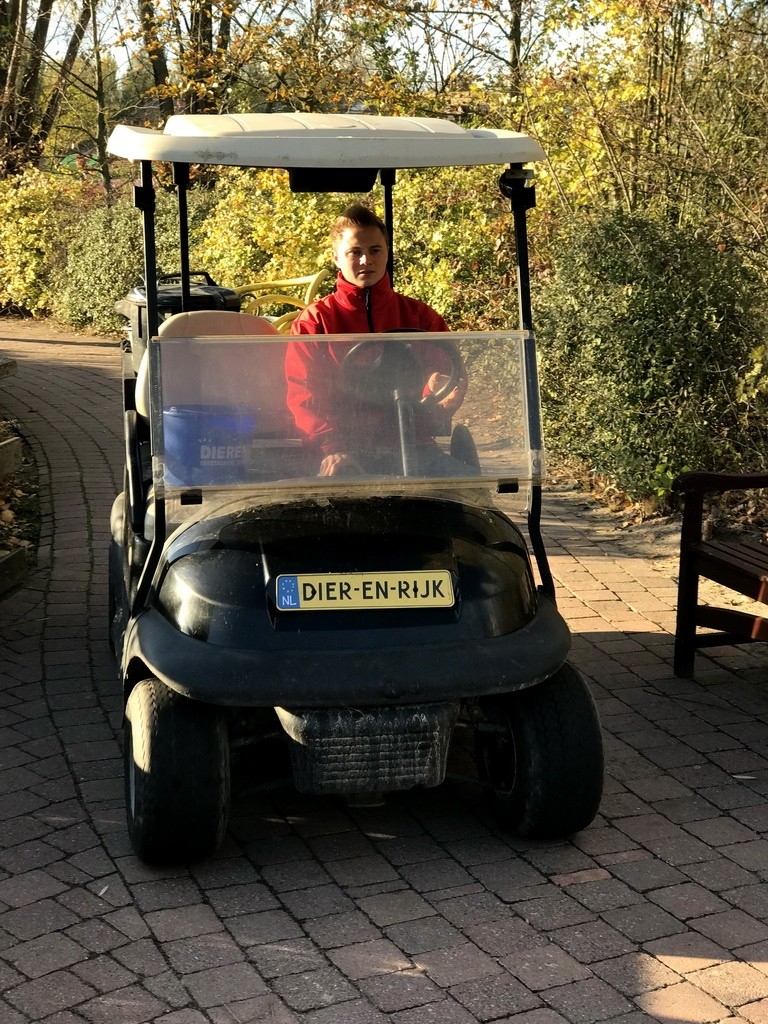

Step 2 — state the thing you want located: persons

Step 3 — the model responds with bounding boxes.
[283,206,467,480]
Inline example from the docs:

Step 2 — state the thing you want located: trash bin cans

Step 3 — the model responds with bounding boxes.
[114,285,241,376]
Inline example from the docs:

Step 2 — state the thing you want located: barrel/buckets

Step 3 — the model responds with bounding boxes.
[162,404,258,485]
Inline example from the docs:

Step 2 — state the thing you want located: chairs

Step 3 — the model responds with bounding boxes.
[135,310,288,433]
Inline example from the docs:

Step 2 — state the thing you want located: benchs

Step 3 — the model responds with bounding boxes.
[671,470,768,678]
